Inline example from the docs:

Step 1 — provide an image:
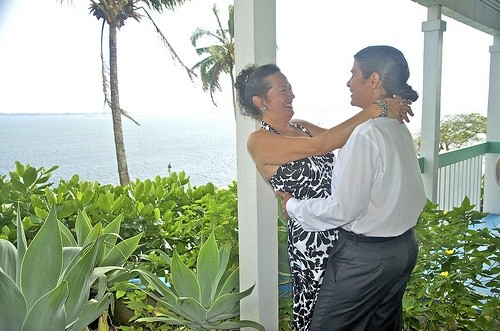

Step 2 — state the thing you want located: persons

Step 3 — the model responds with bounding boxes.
[273,44,428,331]
[232,62,415,331]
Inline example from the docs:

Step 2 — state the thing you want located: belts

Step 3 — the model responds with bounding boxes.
[339,229,396,243]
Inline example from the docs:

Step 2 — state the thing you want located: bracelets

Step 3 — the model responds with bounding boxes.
[373,100,389,117]
